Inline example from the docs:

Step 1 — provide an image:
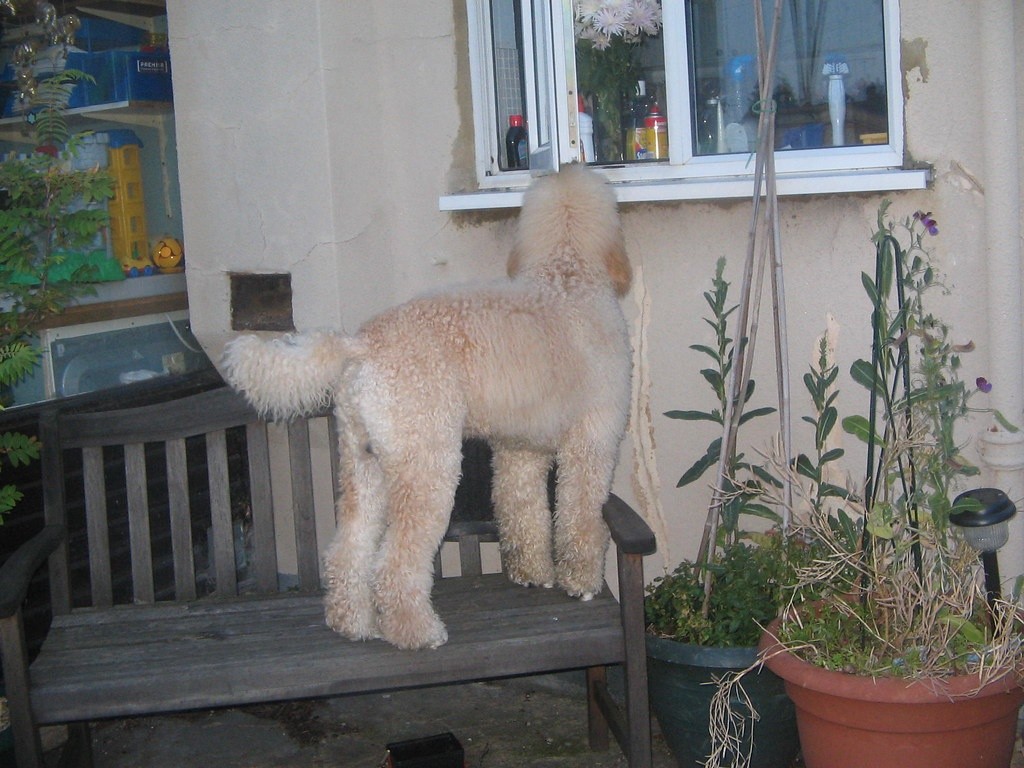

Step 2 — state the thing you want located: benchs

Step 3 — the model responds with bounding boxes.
[0,380,660,768]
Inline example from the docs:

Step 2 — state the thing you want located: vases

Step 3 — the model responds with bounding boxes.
[590,77,623,164]
[756,590,1024,768]
[827,74,846,146]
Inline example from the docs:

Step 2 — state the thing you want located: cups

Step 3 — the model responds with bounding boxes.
[784,124,824,149]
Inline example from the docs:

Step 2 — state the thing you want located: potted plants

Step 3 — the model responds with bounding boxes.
[634,257,807,768]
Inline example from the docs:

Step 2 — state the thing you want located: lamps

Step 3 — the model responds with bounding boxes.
[947,486,1018,642]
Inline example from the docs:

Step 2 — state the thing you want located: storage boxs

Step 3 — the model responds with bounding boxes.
[65,50,174,108]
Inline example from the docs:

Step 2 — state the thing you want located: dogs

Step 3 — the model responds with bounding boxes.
[217,163,635,650]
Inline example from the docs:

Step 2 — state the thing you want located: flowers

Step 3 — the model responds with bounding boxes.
[771,202,1021,679]
[572,0,664,161]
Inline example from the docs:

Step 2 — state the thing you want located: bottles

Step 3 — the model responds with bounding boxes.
[506,56,758,167]
